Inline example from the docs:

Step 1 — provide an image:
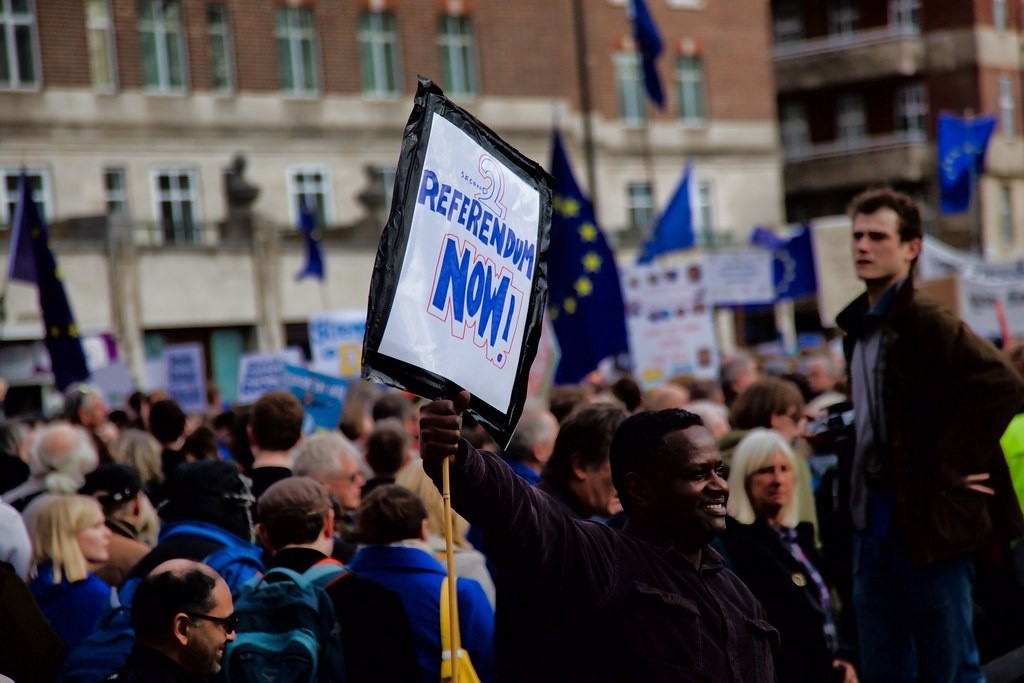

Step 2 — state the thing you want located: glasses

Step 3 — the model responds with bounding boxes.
[784,413,800,424]
[329,470,362,482]
[186,610,238,634]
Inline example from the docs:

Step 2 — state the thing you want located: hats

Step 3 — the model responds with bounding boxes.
[84,463,143,508]
[258,475,334,524]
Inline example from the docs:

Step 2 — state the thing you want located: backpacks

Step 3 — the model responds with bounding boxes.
[222,557,353,683]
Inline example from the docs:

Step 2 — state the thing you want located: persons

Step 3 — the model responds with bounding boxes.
[418,391,774,683]
[0,354,1024,683]
[836,189,1024,683]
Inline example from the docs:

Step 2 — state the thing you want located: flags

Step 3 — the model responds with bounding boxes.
[295,209,322,279]
[5,171,91,394]
[742,227,816,310]
[628,0,665,107]
[639,176,694,264]
[932,114,996,212]
[543,133,630,383]
